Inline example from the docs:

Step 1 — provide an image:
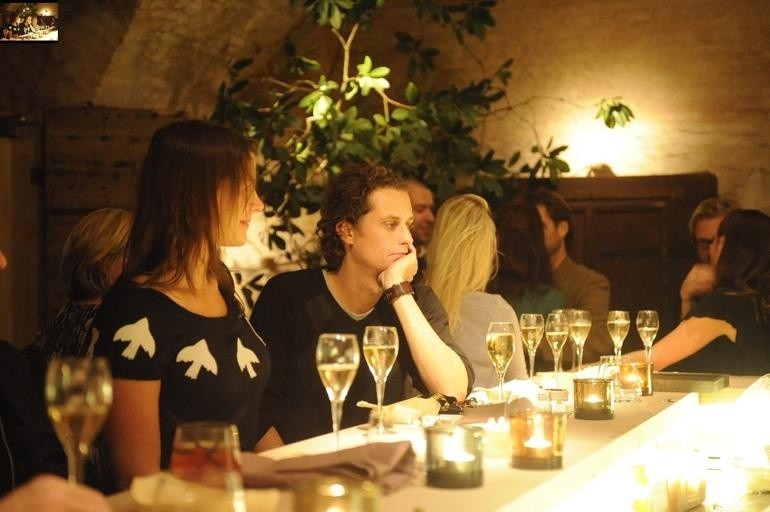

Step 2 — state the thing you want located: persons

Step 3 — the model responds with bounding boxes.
[417,187,612,391]
[0,13,58,41]
[621,197,769,388]
[1,120,476,512]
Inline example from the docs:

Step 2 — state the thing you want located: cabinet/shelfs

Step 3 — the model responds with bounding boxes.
[497,171,718,355]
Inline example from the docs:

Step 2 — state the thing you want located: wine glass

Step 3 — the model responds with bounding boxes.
[519,313,544,390]
[44,355,114,486]
[315,333,360,451]
[485,321,516,403]
[636,310,659,359]
[362,326,399,439]
[606,310,631,358]
[545,308,592,392]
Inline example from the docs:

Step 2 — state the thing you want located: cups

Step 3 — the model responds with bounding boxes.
[170,421,244,495]
[292,357,654,512]
